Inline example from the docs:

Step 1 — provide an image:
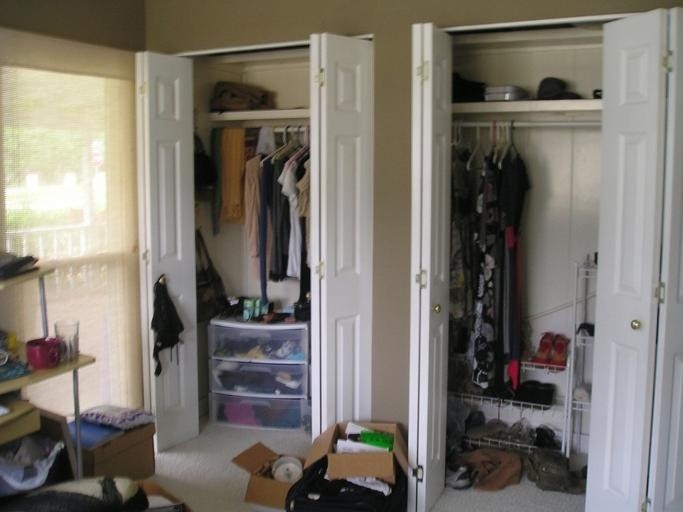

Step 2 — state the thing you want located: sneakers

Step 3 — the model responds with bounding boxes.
[548,334,568,373]
[531,332,554,369]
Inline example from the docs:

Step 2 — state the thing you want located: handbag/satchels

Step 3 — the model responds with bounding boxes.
[195,228,228,324]
[452,448,522,492]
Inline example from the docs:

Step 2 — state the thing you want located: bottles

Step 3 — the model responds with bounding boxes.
[350,428,395,450]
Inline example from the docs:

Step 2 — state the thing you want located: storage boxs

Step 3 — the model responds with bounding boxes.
[231,443,305,509]
[68,419,155,482]
[304,421,408,486]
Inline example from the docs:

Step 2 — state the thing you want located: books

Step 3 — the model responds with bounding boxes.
[333,421,395,453]
[64,418,125,450]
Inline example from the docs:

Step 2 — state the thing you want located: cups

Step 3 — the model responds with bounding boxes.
[54,319,79,361]
[25,337,65,369]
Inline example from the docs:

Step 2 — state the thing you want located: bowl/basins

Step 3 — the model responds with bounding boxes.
[271,455,304,482]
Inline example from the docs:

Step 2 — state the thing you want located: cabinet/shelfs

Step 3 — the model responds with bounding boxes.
[448,361,571,456]
[0,265,96,479]
[566,263,598,459]
[134,32,374,454]
[207,314,309,433]
[407,7,683,511]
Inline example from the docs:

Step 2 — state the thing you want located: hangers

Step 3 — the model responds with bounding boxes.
[261,125,309,168]
[451,120,519,171]
